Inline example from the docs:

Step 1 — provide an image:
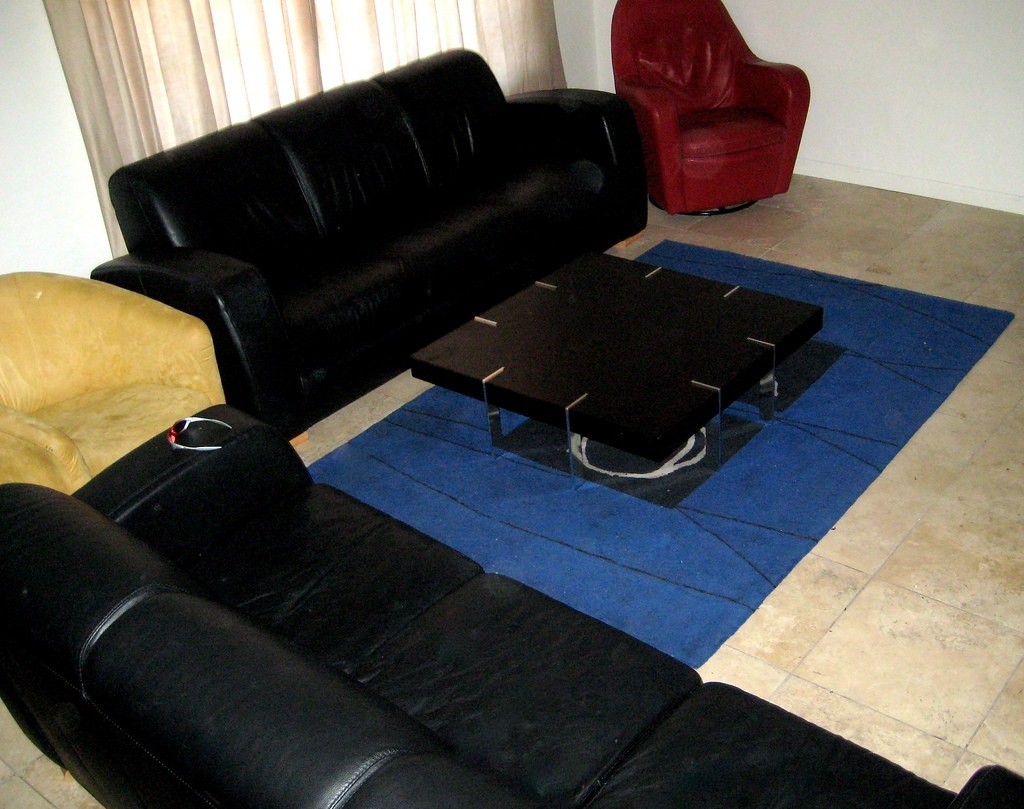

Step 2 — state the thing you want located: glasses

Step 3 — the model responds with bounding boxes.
[167,416,233,453]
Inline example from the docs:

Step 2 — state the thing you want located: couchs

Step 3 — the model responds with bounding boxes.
[93,49,650,443]
[0,271,227,496]
[0,404,1024,809]
[611,0,811,214]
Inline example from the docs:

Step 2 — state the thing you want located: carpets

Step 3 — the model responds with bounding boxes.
[307,236,1015,669]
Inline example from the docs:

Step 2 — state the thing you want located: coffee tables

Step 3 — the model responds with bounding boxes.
[412,251,826,485]
